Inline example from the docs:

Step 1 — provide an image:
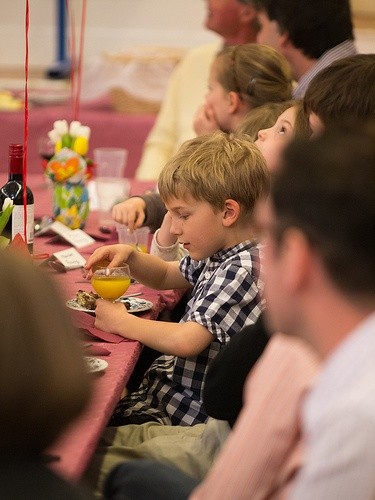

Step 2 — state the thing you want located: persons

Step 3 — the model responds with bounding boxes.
[110,102,310,235]
[302,54,375,128]
[191,334,319,499]
[258,129,374,500]
[134,0,256,180]
[194,43,292,134]
[255,0,356,101]
[85,132,266,426]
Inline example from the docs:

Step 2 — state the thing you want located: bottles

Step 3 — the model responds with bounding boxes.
[0,144,34,253]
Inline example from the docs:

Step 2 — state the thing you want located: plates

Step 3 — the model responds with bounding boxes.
[66,294,153,313]
[80,356,108,372]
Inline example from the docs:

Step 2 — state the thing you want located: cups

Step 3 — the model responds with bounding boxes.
[94,148,127,209]
[95,178,129,234]
[91,262,131,303]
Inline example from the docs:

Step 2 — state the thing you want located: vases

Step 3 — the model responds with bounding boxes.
[50,181,91,231]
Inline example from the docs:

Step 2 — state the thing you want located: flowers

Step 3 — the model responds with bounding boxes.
[0,197,15,250]
[44,119,92,187]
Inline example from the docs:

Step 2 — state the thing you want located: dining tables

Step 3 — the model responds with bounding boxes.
[0,171,177,487]
